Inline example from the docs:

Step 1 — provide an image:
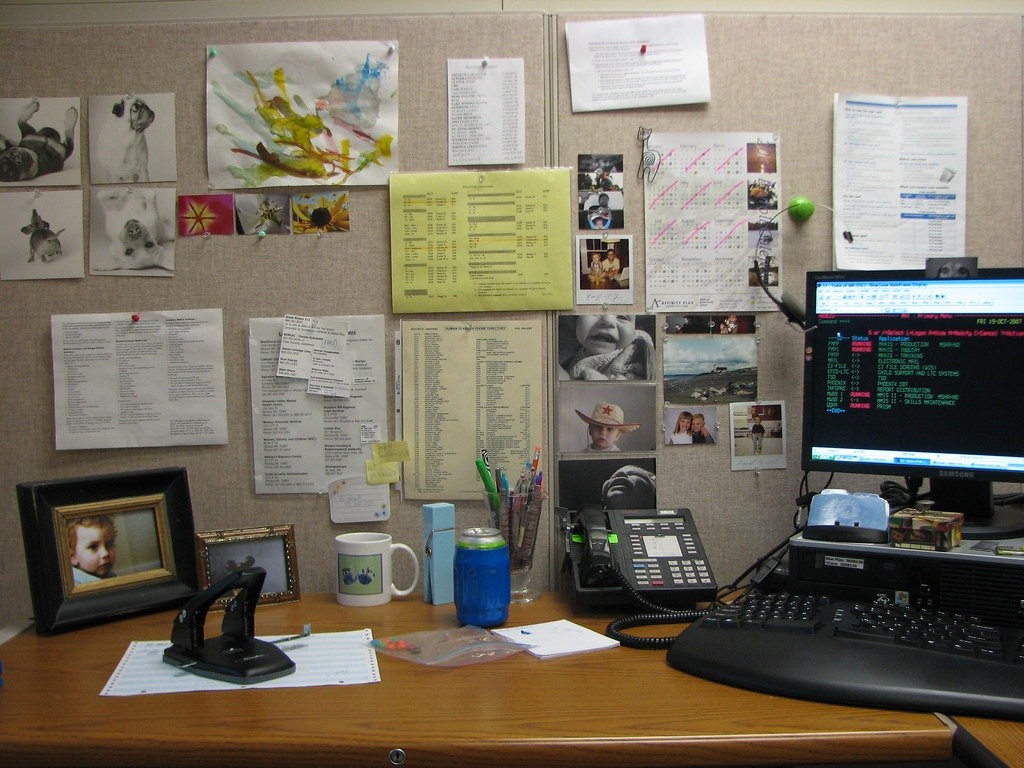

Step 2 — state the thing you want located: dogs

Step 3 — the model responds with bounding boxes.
[92,186,174,271]
[0,100,78,184]
[20,209,66,263]
[95,95,155,183]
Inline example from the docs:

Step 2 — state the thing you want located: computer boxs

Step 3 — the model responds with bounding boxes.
[788,529,1024,634]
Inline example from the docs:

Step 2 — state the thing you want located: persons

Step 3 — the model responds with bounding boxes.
[68,515,116,587]
[570,164,766,511]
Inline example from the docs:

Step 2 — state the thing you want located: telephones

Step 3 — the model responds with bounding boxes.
[559,507,720,617]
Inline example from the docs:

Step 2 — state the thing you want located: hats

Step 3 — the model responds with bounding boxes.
[575,402,641,433]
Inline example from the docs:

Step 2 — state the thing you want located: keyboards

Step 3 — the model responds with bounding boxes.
[666,592,1024,720]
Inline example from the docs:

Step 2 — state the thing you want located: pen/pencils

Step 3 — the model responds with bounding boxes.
[475,446,544,571]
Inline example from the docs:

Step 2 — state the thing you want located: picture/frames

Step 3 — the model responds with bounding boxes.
[15,467,202,639]
[196,522,302,614]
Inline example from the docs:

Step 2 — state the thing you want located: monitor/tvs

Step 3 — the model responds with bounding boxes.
[802,270,1024,540]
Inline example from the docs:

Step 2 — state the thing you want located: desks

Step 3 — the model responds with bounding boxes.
[0,588,1024,768]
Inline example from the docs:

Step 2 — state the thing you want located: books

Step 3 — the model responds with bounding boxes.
[491,620,620,661]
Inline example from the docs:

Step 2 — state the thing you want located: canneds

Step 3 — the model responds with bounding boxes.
[456,528,505,548]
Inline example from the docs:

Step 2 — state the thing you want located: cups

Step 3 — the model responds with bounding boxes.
[334,532,419,608]
[483,489,546,603]
